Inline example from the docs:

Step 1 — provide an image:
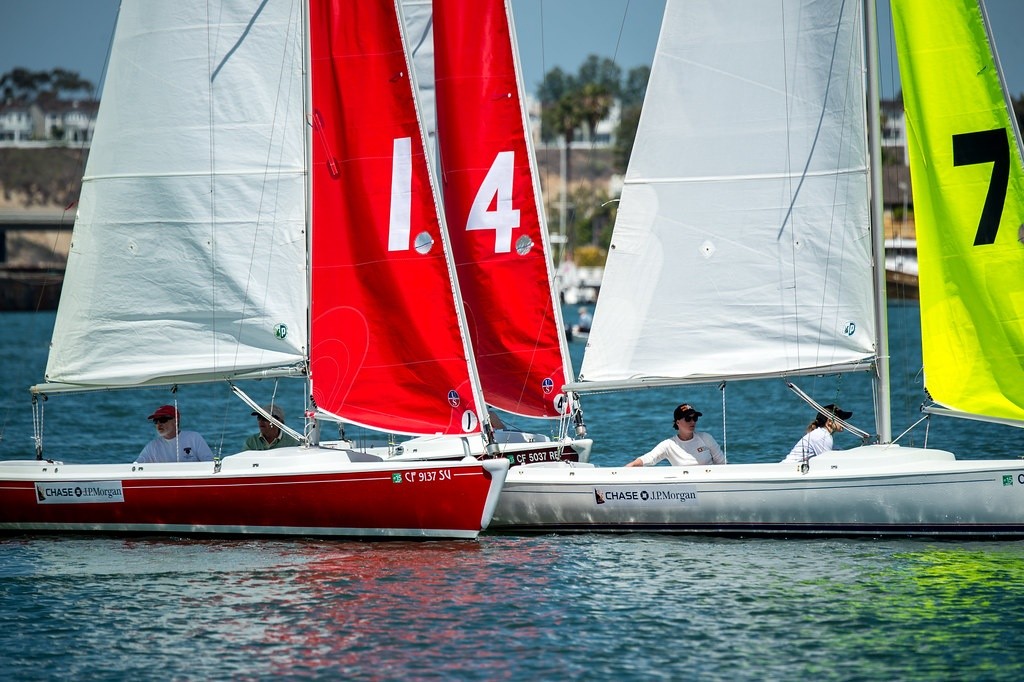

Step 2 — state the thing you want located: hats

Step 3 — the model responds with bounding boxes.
[251,404,284,423]
[816,404,852,427]
[148,405,179,419]
[674,404,702,420]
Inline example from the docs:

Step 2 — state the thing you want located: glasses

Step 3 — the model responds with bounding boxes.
[685,415,698,422]
[153,416,173,424]
[257,417,267,421]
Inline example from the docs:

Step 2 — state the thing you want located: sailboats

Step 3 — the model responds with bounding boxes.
[488,1,1024,534]
[544,132,601,305]
[0,1,507,546]
[316,1,592,463]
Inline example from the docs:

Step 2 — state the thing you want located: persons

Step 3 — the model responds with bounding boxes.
[240,405,300,454]
[135,405,213,463]
[781,404,853,463]
[626,404,728,468]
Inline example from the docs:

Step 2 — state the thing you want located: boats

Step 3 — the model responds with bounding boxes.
[565,323,588,344]
[885,239,919,296]
